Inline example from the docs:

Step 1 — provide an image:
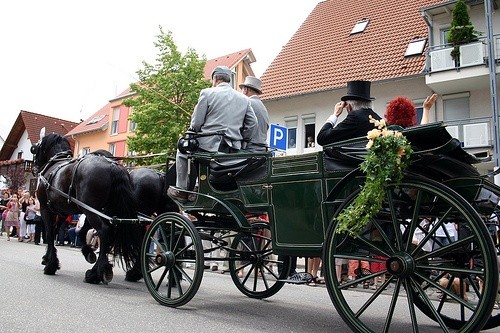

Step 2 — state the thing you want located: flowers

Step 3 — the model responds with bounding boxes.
[330,114,414,240]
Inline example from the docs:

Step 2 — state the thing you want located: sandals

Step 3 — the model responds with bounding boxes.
[313,276,325,284]
[306,278,316,286]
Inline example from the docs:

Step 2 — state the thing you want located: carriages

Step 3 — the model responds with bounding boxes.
[30,120,500,333]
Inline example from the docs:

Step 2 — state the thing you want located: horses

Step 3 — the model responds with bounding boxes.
[83,150,187,287]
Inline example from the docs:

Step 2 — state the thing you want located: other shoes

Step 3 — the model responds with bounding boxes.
[169,187,188,203]
[363,282,370,289]
[371,284,377,289]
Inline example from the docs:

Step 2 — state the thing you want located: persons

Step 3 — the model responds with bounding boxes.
[277,255,389,290]
[383,93,438,127]
[0,191,101,253]
[288,139,295,148]
[428,217,428,219]
[317,80,386,146]
[307,137,315,147]
[238,75,270,149]
[438,258,473,301]
[400,218,457,252]
[146,210,273,278]
[166,65,259,204]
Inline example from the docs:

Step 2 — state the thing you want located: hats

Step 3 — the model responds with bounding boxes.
[341,80,376,102]
[239,75,263,95]
[211,65,231,79]
